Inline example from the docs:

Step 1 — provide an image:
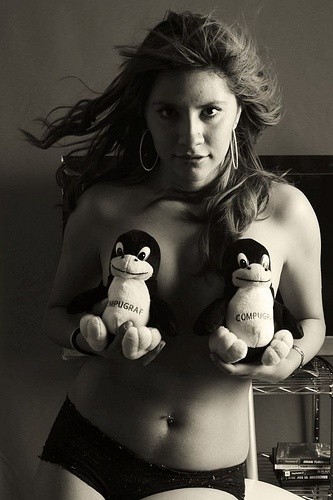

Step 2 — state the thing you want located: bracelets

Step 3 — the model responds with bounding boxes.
[289,344,304,378]
[70,328,94,356]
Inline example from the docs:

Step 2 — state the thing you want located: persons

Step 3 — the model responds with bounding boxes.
[19,11,326,500]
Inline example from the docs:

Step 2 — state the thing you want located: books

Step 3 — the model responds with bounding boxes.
[272,441,332,487]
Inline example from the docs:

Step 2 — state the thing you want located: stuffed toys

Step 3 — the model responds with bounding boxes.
[67,230,177,360]
[193,238,304,366]
